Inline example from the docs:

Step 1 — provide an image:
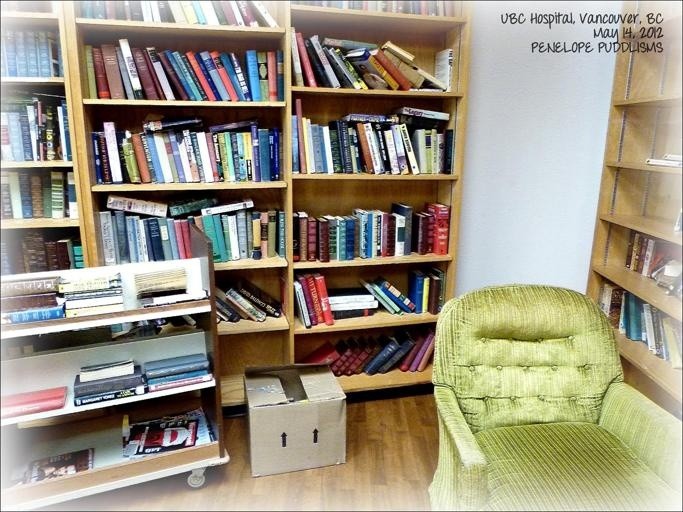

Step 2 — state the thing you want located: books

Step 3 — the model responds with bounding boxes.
[1,1,462,493]
[600,151,683,373]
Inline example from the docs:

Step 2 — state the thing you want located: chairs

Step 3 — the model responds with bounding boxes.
[426,285,683,512]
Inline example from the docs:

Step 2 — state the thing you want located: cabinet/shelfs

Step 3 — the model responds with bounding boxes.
[286,0,473,395]
[0,223,231,512]
[0,0,91,269]
[586,1,683,426]
[61,1,294,409]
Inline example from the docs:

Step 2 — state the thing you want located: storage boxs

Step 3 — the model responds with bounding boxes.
[243,360,347,478]
